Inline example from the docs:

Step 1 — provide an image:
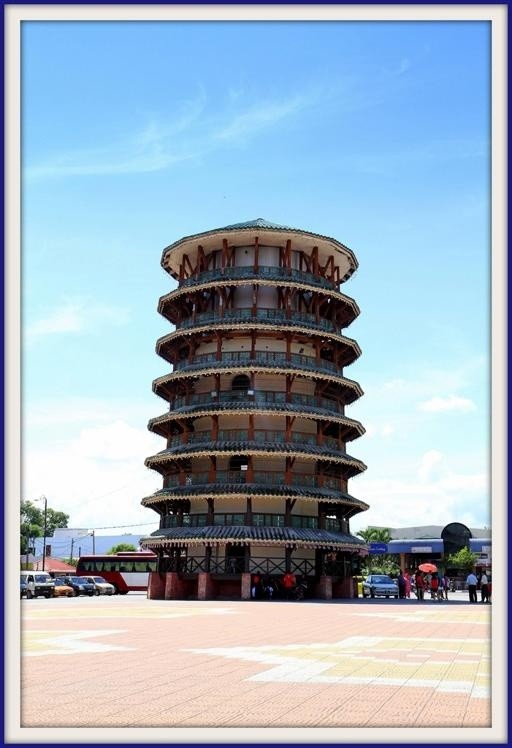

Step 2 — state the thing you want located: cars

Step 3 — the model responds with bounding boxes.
[20,571,115,599]
[362,575,399,599]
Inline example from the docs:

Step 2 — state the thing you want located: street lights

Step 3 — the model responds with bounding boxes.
[34,496,47,571]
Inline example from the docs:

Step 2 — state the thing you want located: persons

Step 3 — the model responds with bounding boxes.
[250,561,490,604]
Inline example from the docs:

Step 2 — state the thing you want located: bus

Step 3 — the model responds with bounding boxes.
[76,552,186,595]
